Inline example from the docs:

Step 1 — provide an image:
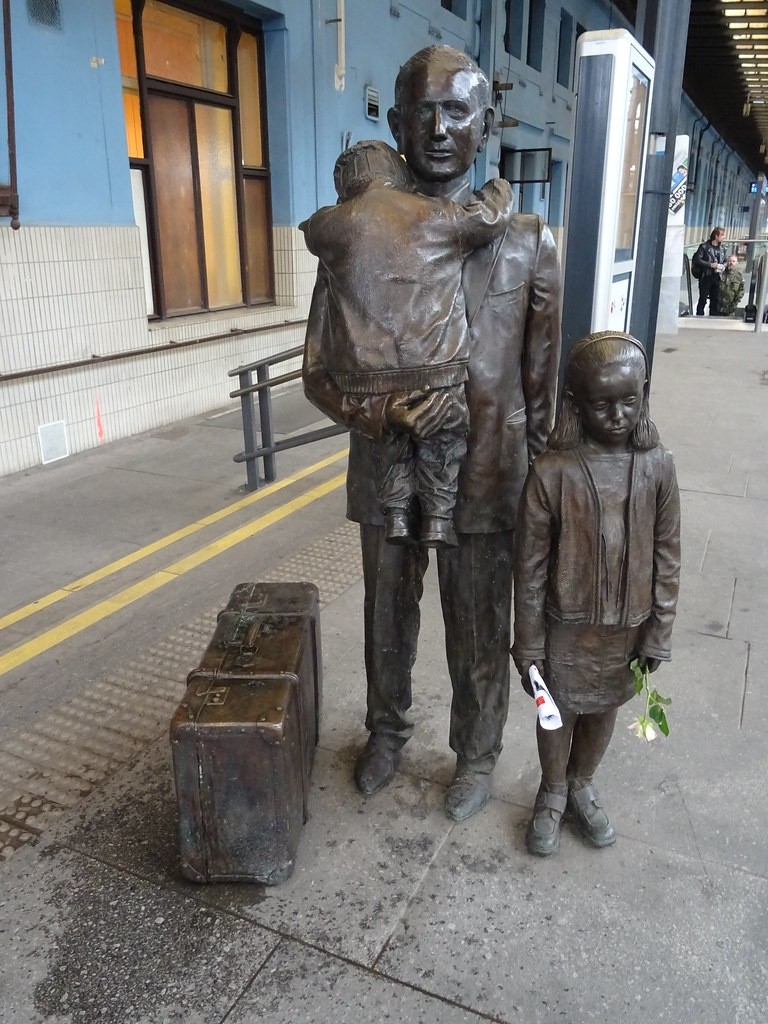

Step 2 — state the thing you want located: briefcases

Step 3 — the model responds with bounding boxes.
[170,580,324,890]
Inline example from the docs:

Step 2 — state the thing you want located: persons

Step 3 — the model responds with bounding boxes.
[301,141,514,546]
[695,228,728,316]
[677,164,687,176]
[304,45,564,822]
[720,256,745,315]
[504,333,680,855]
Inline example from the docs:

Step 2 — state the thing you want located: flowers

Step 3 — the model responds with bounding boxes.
[627,656,674,743]
